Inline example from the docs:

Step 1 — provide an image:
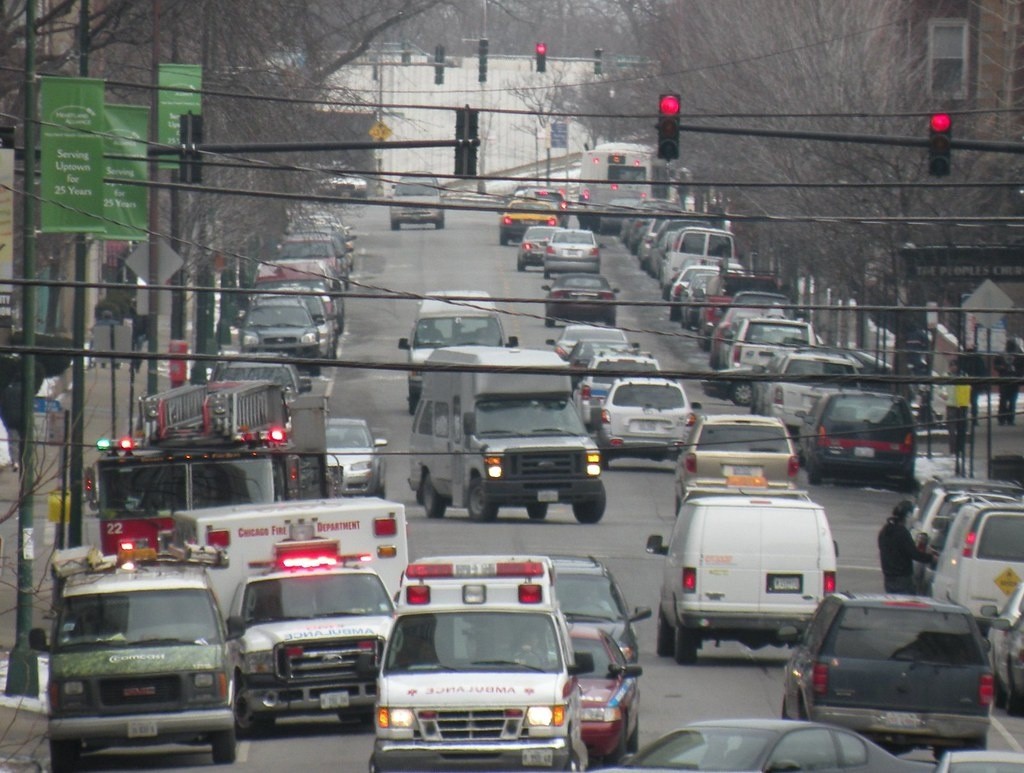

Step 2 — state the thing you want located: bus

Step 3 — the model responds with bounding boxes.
[577,143,670,236]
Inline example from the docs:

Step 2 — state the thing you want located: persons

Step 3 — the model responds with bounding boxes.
[878,500,936,597]
[956,343,985,426]
[994,340,1024,425]
[941,360,972,453]
[578,588,611,612]
[128,299,148,375]
[513,629,549,670]
[95,311,121,369]
[0,372,22,472]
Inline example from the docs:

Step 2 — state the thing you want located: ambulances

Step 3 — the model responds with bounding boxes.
[368,553,595,772]
[171,494,410,739]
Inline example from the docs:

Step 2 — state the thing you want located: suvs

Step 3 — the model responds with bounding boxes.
[908,473,1024,638]
[778,591,995,759]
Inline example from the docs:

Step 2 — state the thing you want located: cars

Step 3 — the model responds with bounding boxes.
[550,556,652,664]
[568,625,643,767]
[588,719,1023,773]
[980,581,1024,719]
[326,418,388,500]
[212,167,445,500]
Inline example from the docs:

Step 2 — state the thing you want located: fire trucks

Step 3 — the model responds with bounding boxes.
[85,379,344,569]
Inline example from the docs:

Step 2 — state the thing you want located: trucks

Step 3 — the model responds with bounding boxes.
[407,345,607,524]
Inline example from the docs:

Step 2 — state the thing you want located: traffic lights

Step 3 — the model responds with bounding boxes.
[928,112,951,176]
[498,186,917,516]
[537,43,546,72]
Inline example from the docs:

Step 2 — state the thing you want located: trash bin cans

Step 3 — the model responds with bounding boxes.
[990,453,1024,484]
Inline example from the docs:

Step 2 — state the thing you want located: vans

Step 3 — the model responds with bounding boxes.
[645,476,840,665]
[397,289,519,417]
[27,562,247,773]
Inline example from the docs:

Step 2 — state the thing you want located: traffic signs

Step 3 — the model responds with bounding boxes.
[658,94,679,160]
